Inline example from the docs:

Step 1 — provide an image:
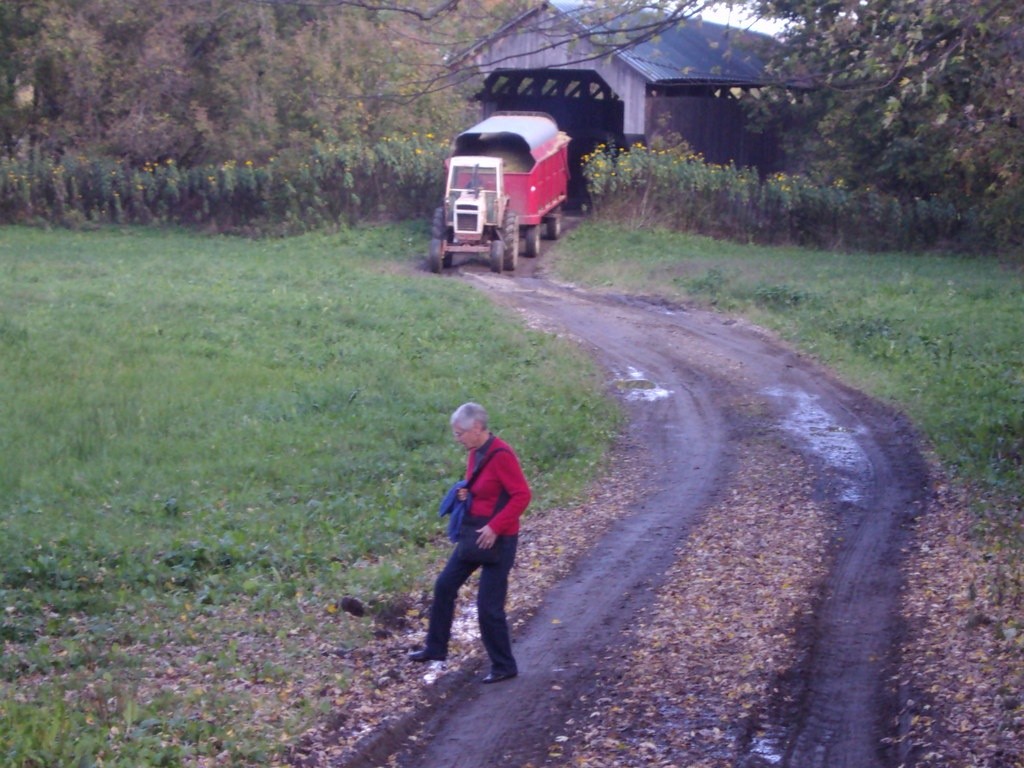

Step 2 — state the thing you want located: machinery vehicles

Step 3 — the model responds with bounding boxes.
[430,110,570,274]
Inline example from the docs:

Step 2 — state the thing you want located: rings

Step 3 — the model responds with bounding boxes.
[486,540,490,544]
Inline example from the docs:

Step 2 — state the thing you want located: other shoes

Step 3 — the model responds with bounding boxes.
[409,652,445,662]
[482,674,517,682]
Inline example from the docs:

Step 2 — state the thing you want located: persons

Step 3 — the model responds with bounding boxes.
[408,402,533,683]
